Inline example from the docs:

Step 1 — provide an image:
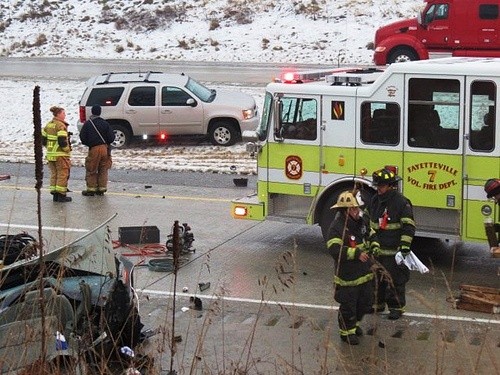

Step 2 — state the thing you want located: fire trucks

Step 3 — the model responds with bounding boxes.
[230,57,500,247]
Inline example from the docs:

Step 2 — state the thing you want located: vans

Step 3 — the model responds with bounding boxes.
[76,71,261,149]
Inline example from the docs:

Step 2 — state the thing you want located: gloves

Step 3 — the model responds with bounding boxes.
[398,241,411,259]
[371,243,382,257]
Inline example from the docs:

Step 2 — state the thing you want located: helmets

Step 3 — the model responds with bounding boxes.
[484,179,500,198]
[329,190,365,209]
[372,169,403,186]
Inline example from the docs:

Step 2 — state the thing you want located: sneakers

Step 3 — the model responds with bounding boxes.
[51,192,72,202]
[82,190,96,196]
[388,310,401,320]
[340,334,360,345]
[376,303,385,312]
[96,190,106,195]
[349,325,362,336]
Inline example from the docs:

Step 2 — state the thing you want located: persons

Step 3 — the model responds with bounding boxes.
[79,103,115,196]
[362,168,416,321]
[41,105,72,202]
[326,187,381,346]
[484,178,500,256]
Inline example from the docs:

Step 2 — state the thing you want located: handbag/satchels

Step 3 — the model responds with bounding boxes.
[108,146,111,156]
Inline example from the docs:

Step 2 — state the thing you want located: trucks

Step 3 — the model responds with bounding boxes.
[372,0,500,65]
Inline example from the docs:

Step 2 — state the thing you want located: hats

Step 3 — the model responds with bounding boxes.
[92,104,101,116]
